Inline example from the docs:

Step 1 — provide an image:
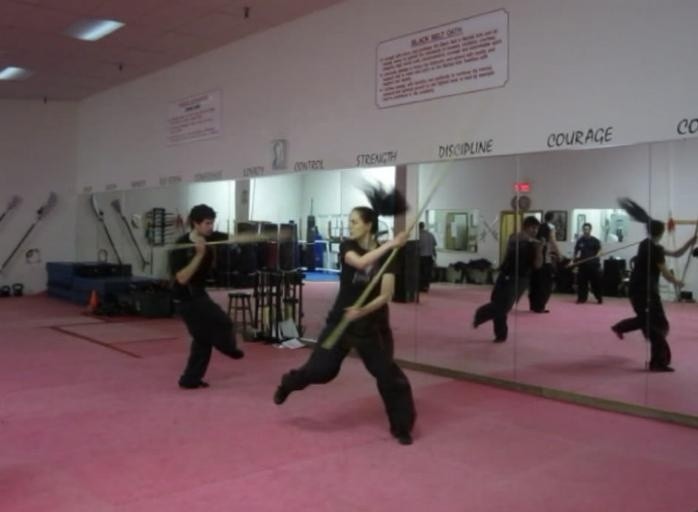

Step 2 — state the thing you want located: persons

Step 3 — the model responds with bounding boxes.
[167,205,244,388]
[473,216,545,343]
[416,221,436,292]
[529,212,561,313]
[274,184,416,444]
[611,198,698,372]
[573,224,603,304]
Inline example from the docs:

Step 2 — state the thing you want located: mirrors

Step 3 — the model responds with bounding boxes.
[78,135,696,430]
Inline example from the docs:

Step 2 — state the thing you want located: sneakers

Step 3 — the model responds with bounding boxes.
[611,325,624,340]
[274,381,292,404]
[390,426,413,445]
[650,362,675,372]
[178,379,210,389]
